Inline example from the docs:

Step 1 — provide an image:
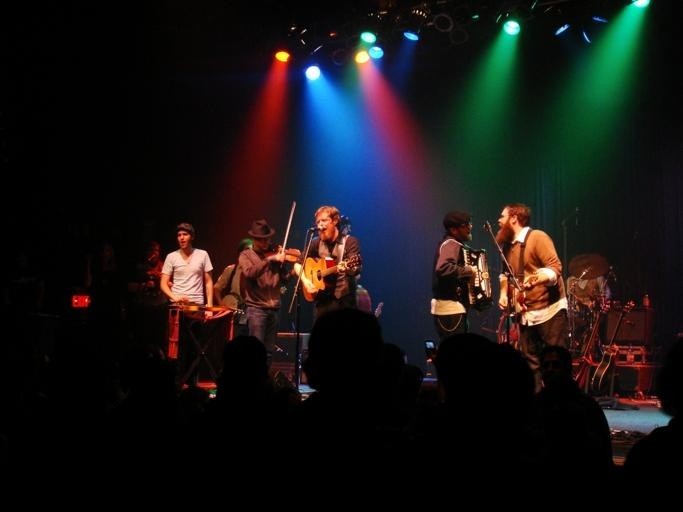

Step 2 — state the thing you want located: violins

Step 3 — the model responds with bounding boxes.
[263,244,302,263]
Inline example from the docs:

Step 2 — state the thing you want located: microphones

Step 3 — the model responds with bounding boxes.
[310,223,319,233]
[482,217,491,232]
[575,205,580,227]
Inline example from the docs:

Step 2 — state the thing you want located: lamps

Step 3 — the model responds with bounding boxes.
[404,17,421,41]
[360,22,378,44]
[350,39,369,64]
[370,44,385,58]
[274,37,292,64]
[502,15,521,36]
[303,57,321,80]
[544,7,569,35]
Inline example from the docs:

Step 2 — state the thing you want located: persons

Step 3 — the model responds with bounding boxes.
[215,240,253,335]
[494,203,571,391]
[297,206,360,328]
[240,220,286,344]
[567,276,612,301]
[430,211,494,340]
[536,345,616,512]
[160,221,212,373]
[126,242,165,333]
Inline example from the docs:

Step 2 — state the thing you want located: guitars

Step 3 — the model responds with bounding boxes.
[591,298,636,396]
[503,273,537,320]
[302,254,361,302]
[571,298,614,396]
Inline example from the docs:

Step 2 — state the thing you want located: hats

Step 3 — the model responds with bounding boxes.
[443,212,471,225]
[247,219,274,237]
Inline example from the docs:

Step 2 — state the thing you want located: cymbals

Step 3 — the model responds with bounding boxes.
[568,252,608,280]
